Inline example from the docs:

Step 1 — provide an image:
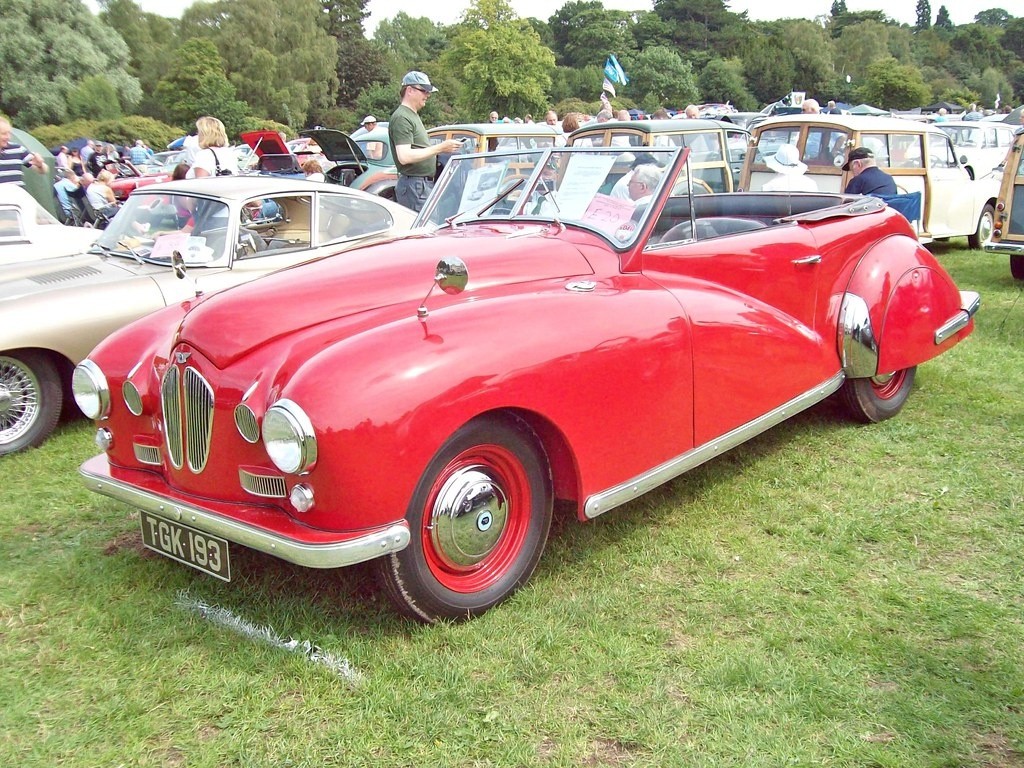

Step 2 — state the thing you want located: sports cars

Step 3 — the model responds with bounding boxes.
[73,148,979,626]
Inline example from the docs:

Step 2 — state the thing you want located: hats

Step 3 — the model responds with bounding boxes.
[842,147,874,171]
[360,116,376,125]
[402,71,439,93]
[632,154,666,171]
[762,144,808,174]
[71,146,79,151]
[95,140,103,146]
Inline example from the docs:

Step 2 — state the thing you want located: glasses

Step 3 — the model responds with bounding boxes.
[411,85,431,96]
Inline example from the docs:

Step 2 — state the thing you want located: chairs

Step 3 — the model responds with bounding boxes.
[660,217,769,249]
[209,234,256,259]
[866,190,921,238]
[64,182,124,229]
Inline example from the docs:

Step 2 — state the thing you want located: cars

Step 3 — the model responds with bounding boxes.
[741,109,1024,256]
[0,176,439,456]
[982,134,1024,279]
[297,129,398,203]
[555,119,752,196]
[427,123,567,185]
[103,146,182,200]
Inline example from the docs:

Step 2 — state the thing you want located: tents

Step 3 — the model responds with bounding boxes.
[848,104,890,116]
[628,109,643,115]
[49,136,124,156]
[821,102,852,113]
[166,137,185,148]
[921,102,962,115]
[8,127,60,220]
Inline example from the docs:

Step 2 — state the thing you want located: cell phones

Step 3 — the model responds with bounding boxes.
[460,138,467,142]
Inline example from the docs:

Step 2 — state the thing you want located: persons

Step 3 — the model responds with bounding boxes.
[827,101,842,115]
[936,106,1012,116]
[839,146,898,204]
[0,116,49,189]
[488,105,718,151]
[963,104,982,121]
[388,71,465,224]
[300,160,325,183]
[360,117,389,159]
[1019,109,1024,125]
[522,162,559,219]
[795,99,845,165]
[306,138,322,151]
[760,143,822,193]
[610,151,658,200]
[278,132,293,153]
[625,164,662,207]
[185,117,239,232]
[57,138,279,234]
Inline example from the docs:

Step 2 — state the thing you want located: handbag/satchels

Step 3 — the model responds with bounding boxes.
[194,148,232,219]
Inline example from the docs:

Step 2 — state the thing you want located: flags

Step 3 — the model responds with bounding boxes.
[600,92,613,116]
[779,91,806,108]
[604,55,629,85]
[602,78,616,98]
